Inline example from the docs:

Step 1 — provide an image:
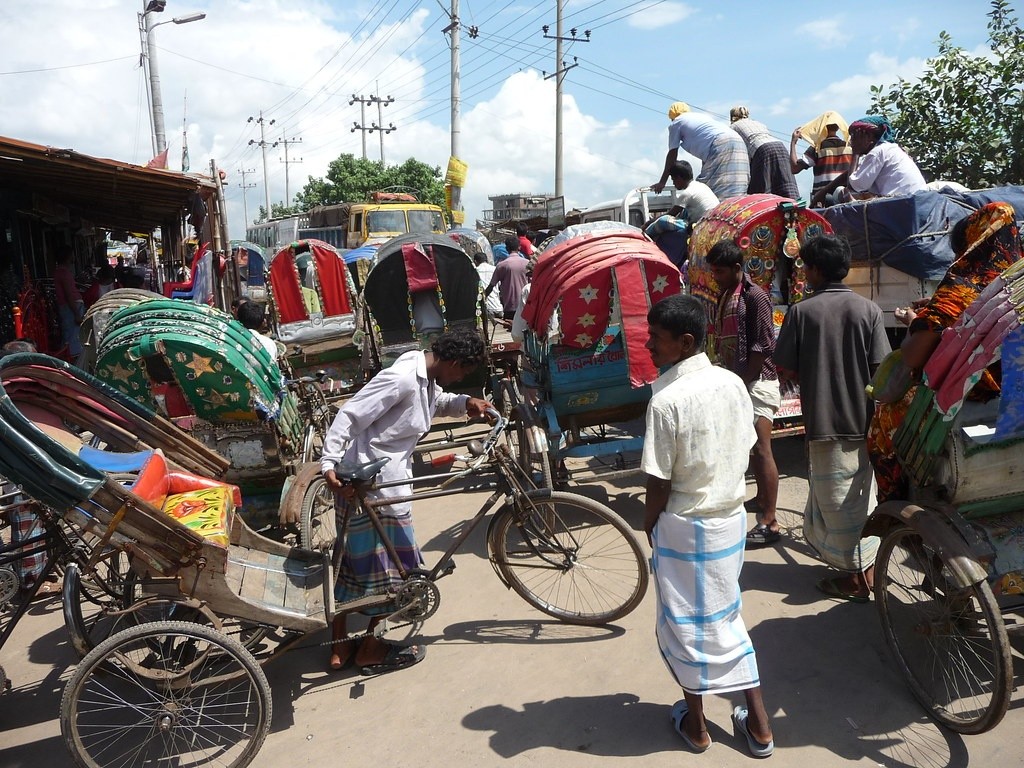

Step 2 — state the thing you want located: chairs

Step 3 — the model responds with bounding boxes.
[126,448,243,546]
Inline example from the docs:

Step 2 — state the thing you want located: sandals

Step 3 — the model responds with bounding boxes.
[746,520,781,544]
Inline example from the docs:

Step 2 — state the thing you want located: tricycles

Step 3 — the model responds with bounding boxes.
[805,263,1023,735]
[0,351,649,767]
[0,193,851,682]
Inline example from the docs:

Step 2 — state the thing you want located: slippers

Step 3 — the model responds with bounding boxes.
[363,643,426,674]
[332,637,358,671]
[816,576,869,602]
[671,699,712,751]
[734,706,774,757]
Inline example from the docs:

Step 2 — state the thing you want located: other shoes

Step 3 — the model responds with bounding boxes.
[30,578,63,602]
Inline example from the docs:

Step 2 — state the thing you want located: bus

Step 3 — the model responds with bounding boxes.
[245,217,310,284]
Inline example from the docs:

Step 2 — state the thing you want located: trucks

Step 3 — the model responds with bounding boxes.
[581,185,1024,327]
[299,186,443,257]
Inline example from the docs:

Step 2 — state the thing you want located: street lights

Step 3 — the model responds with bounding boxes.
[138,0,206,280]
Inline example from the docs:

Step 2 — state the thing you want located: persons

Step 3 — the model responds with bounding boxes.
[50,253,164,360]
[706,240,780,550]
[229,295,277,368]
[472,224,554,394]
[775,204,1024,601]
[640,101,929,238]
[320,327,499,676]
[640,294,774,758]
